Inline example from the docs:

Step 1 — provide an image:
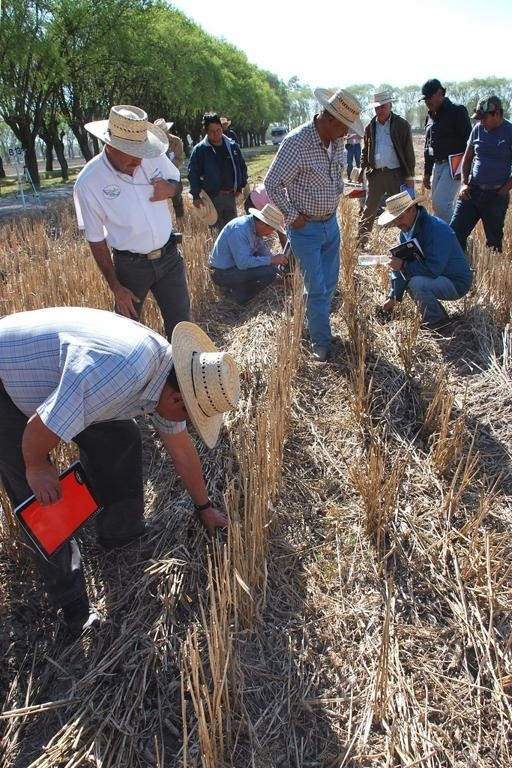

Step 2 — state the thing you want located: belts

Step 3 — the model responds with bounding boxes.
[304,214,332,221]
[435,159,448,164]
[114,236,174,260]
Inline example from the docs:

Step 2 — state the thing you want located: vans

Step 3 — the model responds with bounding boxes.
[271,127,292,145]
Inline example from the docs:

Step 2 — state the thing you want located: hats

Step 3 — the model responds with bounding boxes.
[171,321,239,449]
[248,203,286,235]
[378,190,425,226]
[315,89,364,137]
[193,190,219,226]
[471,95,500,120]
[83,105,169,159]
[220,117,231,126]
[154,118,173,131]
[418,79,440,101]
[369,91,395,107]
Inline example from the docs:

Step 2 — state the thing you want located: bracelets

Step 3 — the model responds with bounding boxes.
[194,497,212,510]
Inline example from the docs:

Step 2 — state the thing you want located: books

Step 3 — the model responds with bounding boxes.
[400,181,415,200]
[448,152,473,179]
[390,238,424,280]
[13,460,104,561]
[345,189,365,197]
[278,240,296,278]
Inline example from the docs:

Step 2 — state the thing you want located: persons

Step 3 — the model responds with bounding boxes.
[265,87,364,361]
[1,306,239,637]
[377,190,472,331]
[356,91,415,250]
[349,166,415,217]
[208,204,289,306]
[73,105,192,342]
[449,94,511,253]
[153,117,185,218]
[344,129,360,184]
[418,78,475,225]
[187,111,248,238]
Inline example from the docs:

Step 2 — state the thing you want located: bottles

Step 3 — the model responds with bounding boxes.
[358,254,391,266]
